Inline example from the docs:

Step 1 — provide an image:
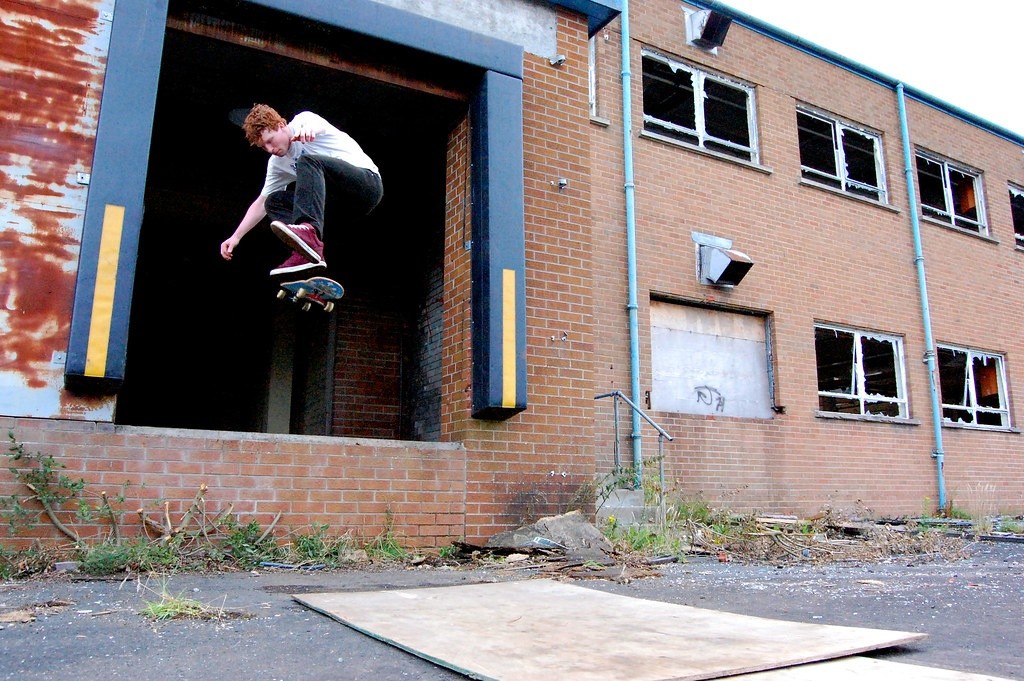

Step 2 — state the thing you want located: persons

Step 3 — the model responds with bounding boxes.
[220,104,382,276]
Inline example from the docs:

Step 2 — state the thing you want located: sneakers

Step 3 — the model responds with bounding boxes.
[270,220,324,264]
[269,251,324,280]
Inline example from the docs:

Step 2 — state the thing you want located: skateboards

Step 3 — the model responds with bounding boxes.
[275,277,345,314]
